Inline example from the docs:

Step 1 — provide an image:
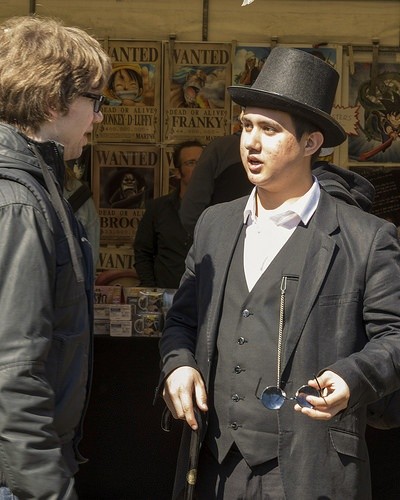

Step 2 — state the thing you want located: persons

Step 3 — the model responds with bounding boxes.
[0,15,111,500]
[158,46,400,500]
[62,131,256,287]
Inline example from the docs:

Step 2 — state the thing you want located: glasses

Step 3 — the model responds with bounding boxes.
[254,374,322,410]
[180,160,198,169]
[75,90,110,113]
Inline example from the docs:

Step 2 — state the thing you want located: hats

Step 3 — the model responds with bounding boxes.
[227,47,347,148]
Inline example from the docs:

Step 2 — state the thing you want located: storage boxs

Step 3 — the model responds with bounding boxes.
[93,304,133,337]
